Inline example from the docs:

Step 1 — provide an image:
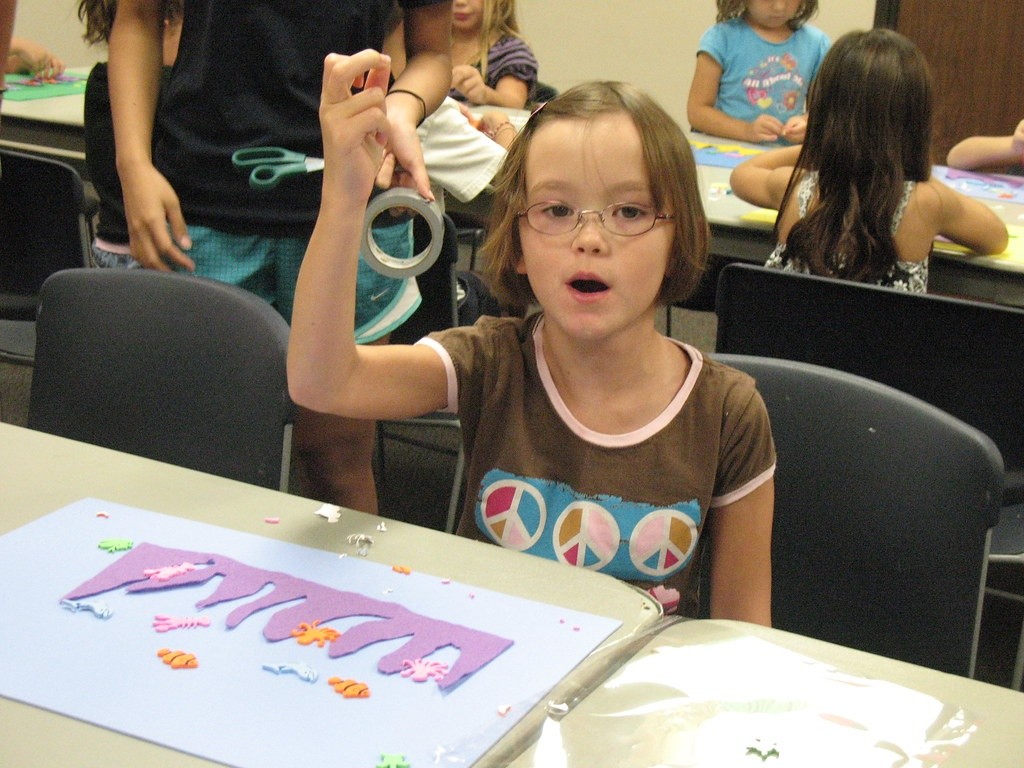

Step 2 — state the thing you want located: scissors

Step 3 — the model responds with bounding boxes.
[233,147,326,193]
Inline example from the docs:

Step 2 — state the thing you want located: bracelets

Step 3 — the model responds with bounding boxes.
[482,120,511,136]
[385,90,426,129]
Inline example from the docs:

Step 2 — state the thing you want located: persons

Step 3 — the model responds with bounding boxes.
[0,0,65,91]
[729,27,1009,298]
[946,119,1024,170]
[686,0,834,144]
[79,1,539,514]
[287,49,777,630]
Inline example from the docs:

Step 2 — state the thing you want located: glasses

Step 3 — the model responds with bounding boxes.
[517,200,677,235]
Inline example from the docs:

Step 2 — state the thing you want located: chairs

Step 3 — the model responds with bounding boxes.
[27,266,297,493]
[713,262,1024,688]
[706,350,1008,679]
[0,147,99,368]
[377,213,501,473]
[454,83,558,270]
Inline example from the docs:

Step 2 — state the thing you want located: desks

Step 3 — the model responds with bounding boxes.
[496,617,1024,768]
[0,420,666,768]
[0,65,1024,308]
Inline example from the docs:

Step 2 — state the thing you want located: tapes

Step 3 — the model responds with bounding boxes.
[360,187,444,279]
